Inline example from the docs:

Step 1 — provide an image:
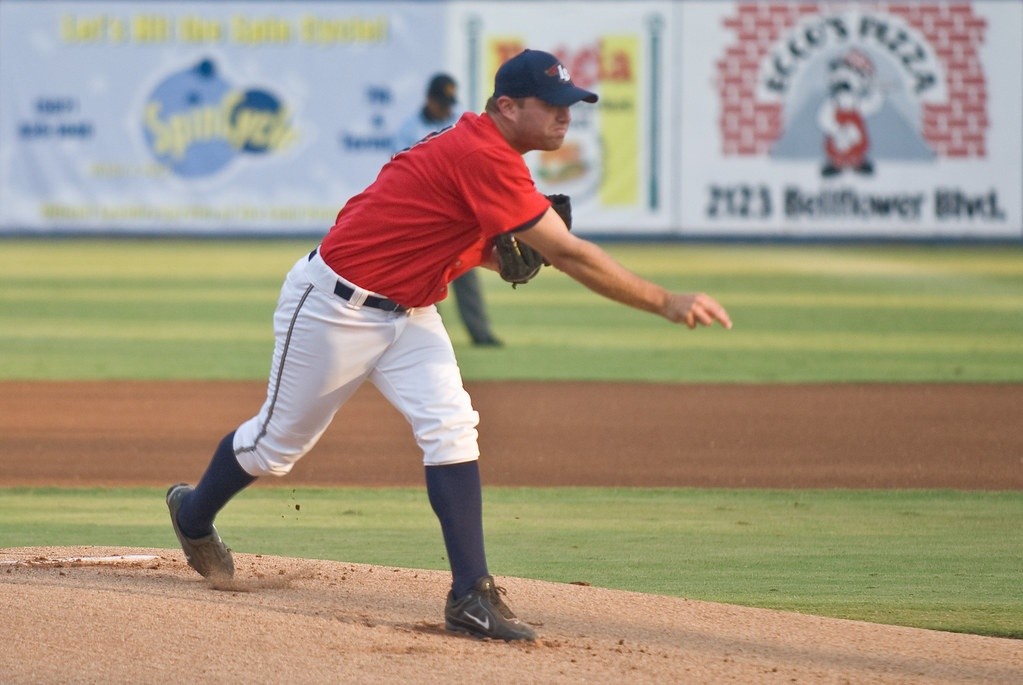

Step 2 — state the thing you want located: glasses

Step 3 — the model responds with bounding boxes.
[438,93,457,105]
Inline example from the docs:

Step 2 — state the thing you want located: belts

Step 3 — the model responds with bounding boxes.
[307,248,411,312]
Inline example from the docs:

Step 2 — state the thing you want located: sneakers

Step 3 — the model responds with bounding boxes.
[444,574,539,643]
[165,481,234,586]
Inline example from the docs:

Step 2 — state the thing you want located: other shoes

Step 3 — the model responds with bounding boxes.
[472,334,504,347]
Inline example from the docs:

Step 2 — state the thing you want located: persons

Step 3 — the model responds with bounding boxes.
[165,47,734,643]
[392,72,507,348]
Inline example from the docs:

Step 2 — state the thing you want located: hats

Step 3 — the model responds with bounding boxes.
[494,47,599,108]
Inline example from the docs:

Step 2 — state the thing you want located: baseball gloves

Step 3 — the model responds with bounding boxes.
[495,193,572,282]
